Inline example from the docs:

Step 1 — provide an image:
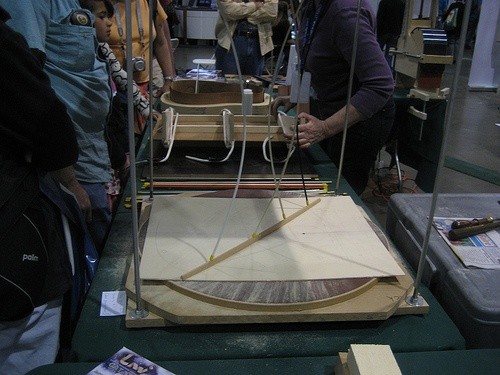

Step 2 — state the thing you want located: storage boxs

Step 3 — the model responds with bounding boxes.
[386,192,500,343]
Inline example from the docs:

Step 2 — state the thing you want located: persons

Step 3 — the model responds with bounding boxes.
[269,0,395,197]
[214,0,278,75]
[0,0,174,375]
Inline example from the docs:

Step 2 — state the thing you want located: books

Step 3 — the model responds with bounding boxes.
[87,347,176,375]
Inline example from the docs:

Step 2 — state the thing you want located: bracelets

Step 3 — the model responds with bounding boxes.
[164,76,173,82]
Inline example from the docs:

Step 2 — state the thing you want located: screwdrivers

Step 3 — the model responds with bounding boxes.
[454,217,500,229]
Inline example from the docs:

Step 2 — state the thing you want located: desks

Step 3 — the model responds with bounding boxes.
[25,88,500,375]
[175,7,219,48]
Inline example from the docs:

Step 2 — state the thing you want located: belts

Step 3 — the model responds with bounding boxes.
[234,31,258,39]
